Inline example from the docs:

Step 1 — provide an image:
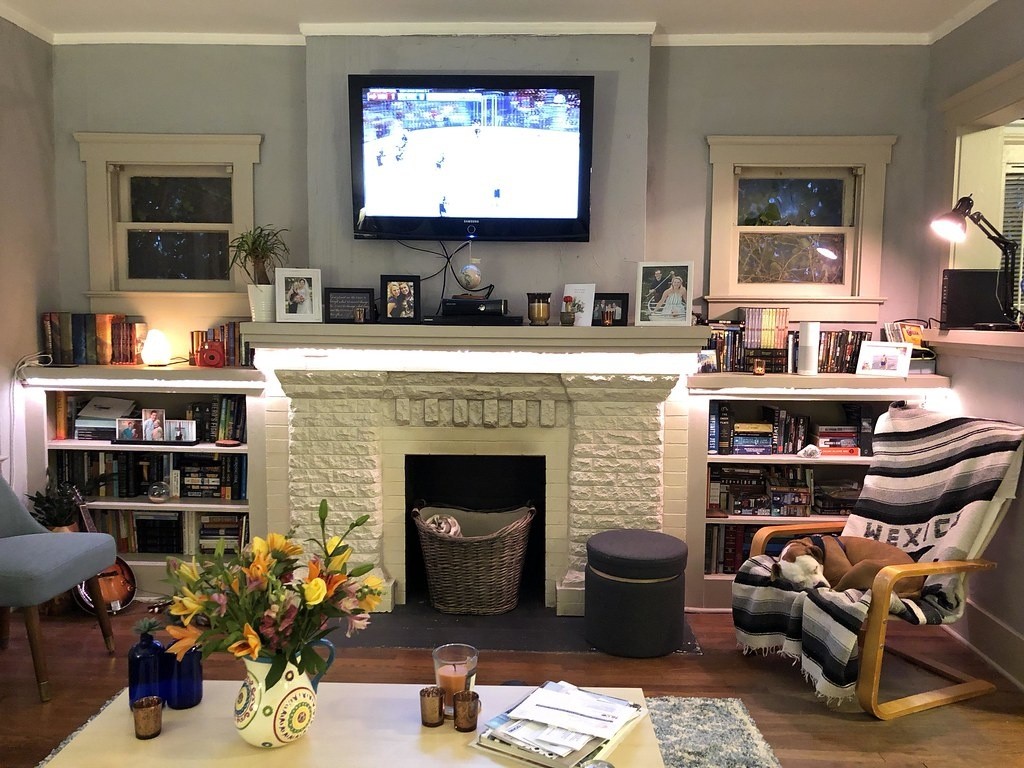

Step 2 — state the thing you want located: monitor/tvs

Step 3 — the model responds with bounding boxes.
[346,75,595,242]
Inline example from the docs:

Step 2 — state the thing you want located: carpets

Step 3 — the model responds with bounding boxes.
[32,687,783,768]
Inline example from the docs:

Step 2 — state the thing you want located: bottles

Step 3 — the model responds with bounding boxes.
[128,634,165,711]
[165,637,203,710]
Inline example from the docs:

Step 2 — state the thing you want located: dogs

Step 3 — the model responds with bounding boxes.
[771,533,925,599]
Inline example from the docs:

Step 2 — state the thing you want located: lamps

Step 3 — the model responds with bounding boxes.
[816,235,842,259]
[930,193,1023,331]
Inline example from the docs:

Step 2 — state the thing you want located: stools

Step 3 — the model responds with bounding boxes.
[585,528,687,658]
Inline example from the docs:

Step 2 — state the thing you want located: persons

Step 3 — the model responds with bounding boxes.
[131,425,137,440]
[144,411,164,441]
[879,355,888,370]
[594,299,621,319]
[288,278,313,314]
[387,282,414,318]
[654,276,687,321]
[359,89,580,219]
[649,269,675,304]
[122,422,133,440]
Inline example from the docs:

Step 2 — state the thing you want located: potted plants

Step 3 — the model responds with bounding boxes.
[226,223,292,323]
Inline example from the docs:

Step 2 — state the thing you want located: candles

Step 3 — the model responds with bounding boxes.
[440,663,474,709]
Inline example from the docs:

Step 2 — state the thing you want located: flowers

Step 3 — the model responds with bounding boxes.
[130,500,386,692]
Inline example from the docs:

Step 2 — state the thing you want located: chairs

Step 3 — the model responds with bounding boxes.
[732,402,1023,719]
[0,473,116,703]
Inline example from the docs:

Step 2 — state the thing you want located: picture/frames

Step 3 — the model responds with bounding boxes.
[141,409,166,441]
[856,341,915,379]
[274,268,323,322]
[116,417,144,442]
[380,274,421,324]
[324,288,375,323]
[634,262,694,326]
[592,292,630,327]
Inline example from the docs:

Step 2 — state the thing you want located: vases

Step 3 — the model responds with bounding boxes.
[232,636,335,749]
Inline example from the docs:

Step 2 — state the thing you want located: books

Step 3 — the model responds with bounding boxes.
[57,451,247,501]
[703,523,794,576]
[706,464,861,517]
[182,511,250,558]
[794,330,872,374]
[708,400,874,457]
[42,311,147,365]
[185,394,247,443]
[54,391,142,440]
[883,322,925,346]
[702,305,799,374]
[467,680,648,768]
[190,321,254,366]
[78,510,182,553]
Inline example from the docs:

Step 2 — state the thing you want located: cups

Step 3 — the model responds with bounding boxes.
[420,686,446,728]
[354,307,365,323]
[526,292,552,325]
[433,643,479,720]
[132,696,163,740]
[601,310,614,326]
[453,690,482,732]
[754,360,766,375]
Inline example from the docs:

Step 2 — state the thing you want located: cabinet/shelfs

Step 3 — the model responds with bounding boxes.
[16,366,268,566]
[688,375,950,610]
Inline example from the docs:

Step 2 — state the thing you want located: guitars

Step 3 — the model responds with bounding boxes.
[60,480,137,617]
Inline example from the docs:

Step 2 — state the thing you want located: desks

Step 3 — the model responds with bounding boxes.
[36,681,667,768]
